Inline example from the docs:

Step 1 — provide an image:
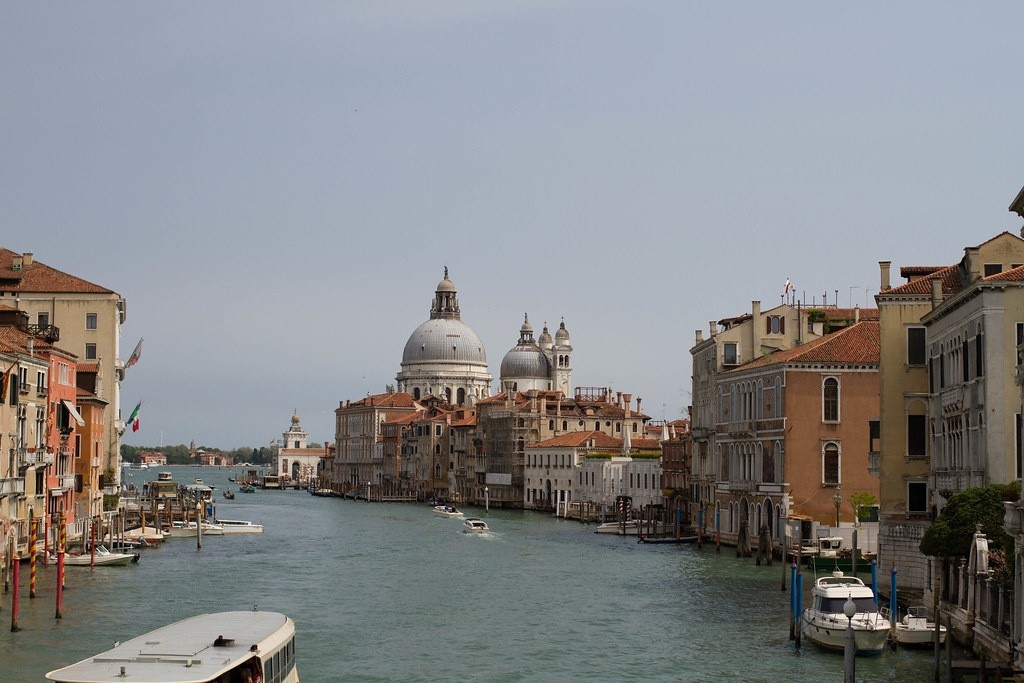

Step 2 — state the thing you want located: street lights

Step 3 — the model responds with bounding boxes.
[833,486,843,527]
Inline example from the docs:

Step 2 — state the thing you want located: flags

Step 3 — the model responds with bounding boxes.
[130,401,142,432]
[128,337,144,369]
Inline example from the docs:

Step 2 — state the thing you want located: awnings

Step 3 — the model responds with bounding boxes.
[62,401,85,427]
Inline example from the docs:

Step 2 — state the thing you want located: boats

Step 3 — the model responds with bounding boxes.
[434,505,465,518]
[37,544,140,566]
[45,607,300,683]
[800,566,892,655]
[891,605,947,645]
[223,490,235,499]
[240,484,256,493]
[801,534,878,572]
[597,520,674,534]
[461,517,490,534]
[102,528,171,549]
[169,518,263,537]
